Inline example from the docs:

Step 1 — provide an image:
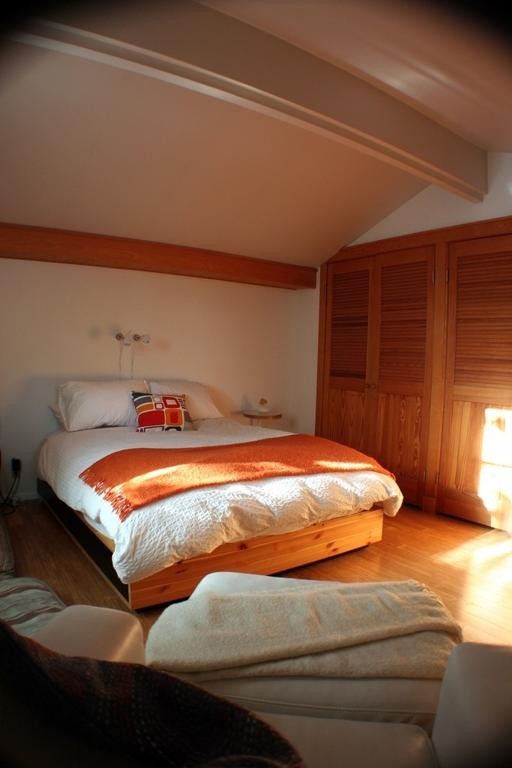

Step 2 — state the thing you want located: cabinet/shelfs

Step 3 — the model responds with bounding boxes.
[439,233,511,536]
[325,247,432,501]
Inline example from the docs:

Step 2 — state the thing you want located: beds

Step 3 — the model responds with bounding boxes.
[36,422,404,614]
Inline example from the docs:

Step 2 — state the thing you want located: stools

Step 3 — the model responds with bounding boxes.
[183,567,440,734]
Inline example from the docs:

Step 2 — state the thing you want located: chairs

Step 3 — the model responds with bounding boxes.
[21,604,512,768]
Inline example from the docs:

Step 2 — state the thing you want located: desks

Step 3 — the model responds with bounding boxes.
[242,410,282,427]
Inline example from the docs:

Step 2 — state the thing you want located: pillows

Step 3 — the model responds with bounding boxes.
[49,374,225,436]
[0,617,305,765]
